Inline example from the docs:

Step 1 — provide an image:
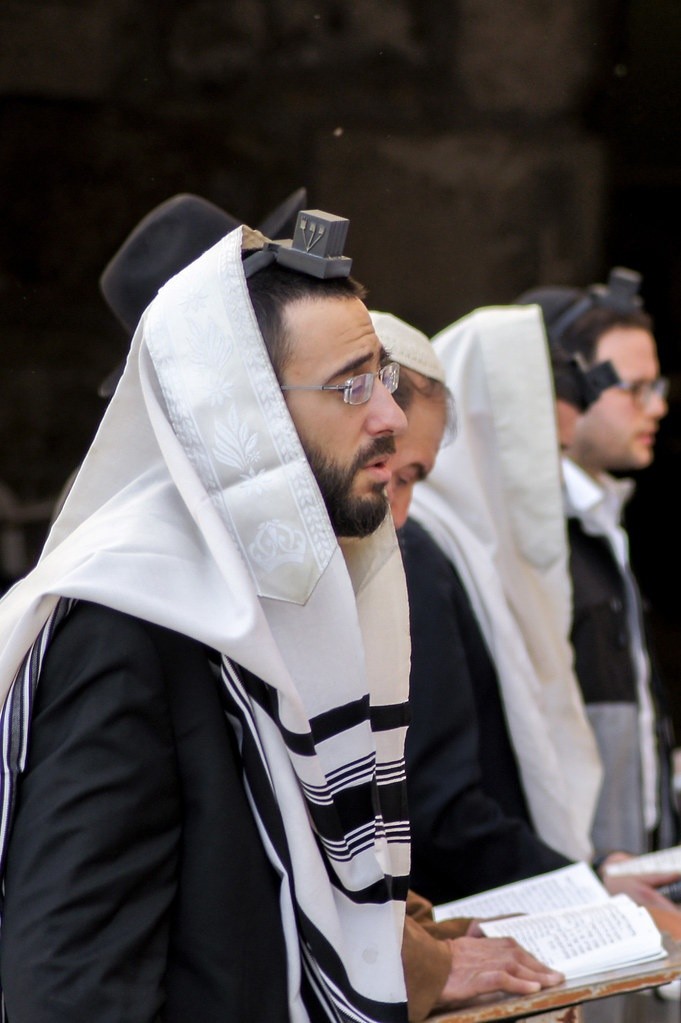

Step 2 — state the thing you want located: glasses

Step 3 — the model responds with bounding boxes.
[279,361,400,406]
[613,379,667,408]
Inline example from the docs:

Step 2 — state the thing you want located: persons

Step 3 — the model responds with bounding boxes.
[0,247,670,1023]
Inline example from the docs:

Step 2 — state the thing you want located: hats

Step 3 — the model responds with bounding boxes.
[98,189,308,397]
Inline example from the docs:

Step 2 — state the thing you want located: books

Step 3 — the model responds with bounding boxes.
[430,860,668,979]
